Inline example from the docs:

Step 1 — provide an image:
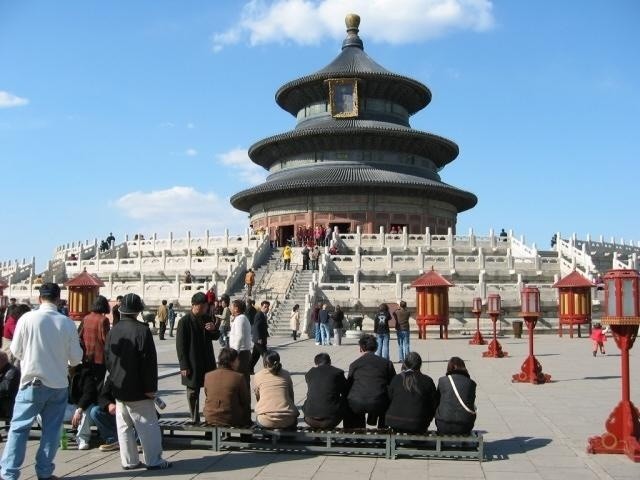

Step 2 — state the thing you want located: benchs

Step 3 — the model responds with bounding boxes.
[1,419,485,462]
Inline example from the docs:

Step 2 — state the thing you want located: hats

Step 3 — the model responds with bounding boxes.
[118,293,144,314]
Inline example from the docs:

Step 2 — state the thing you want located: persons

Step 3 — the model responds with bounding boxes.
[69,253,76,260]
[312,302,343,346]
[551,234,557,247]
[184,271,195,290]
[246,268,255,295]
[374,303,392,359]
[591,323,606,357]
[389,226,396,234]
[595,273,604,291]
[196,246,204,262]
[303,335,477,438]
[133,234,154,245]
[176,287,303,431]
[100,240,108,251]
[397,226,403,234]
[0,283,177,480]
[34,275,43,284]
[107,232,114,247]
[392,301,411,363]
[499,229,507,242]
[247,223,339,270]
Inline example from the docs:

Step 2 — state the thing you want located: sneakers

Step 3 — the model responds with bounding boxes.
[147,461,172,470]
[78,443,89,450]
[99,442,120,451]
